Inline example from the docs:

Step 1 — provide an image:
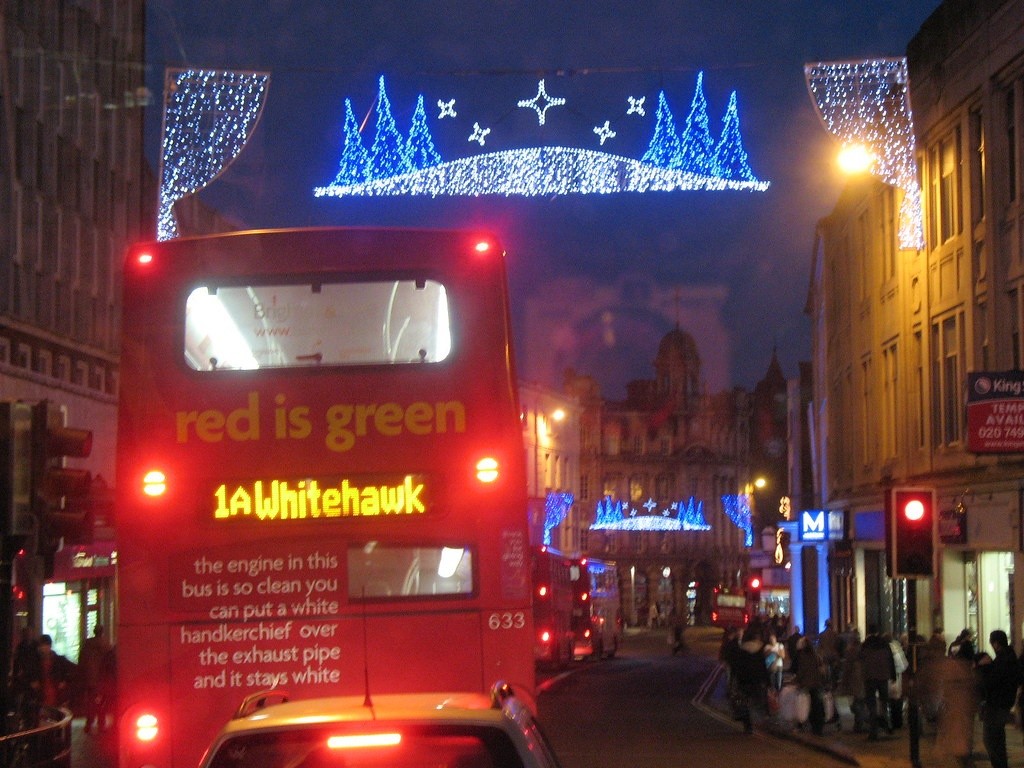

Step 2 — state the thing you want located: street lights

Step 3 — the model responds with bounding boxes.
[533,398,567,549]
[737,478,767,588]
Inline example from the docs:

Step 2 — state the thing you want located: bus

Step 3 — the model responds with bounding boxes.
[109,226,540,768]
[537,544,624,675]
[713,585,755,629]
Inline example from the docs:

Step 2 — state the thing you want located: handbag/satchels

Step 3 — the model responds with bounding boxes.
[888,676,901,699]
[821,690,834,724]
[767,690,779,715]
[732,693,749,723]
[764,654,776,668]
[780,684,811,724]
[833,684,856,717]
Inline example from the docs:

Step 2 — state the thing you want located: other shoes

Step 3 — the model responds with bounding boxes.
[833,723,841,732]
[867,734,876,742]
[879,720,891,734]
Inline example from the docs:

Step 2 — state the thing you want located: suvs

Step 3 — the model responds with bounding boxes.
[196,680,561,768]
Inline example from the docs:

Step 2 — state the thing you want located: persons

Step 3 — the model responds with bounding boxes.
[720,614,1024,768]
[9,622,117,735]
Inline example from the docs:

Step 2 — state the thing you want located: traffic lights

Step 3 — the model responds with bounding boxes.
[26,399,95,556]
[891,483,939,581]
